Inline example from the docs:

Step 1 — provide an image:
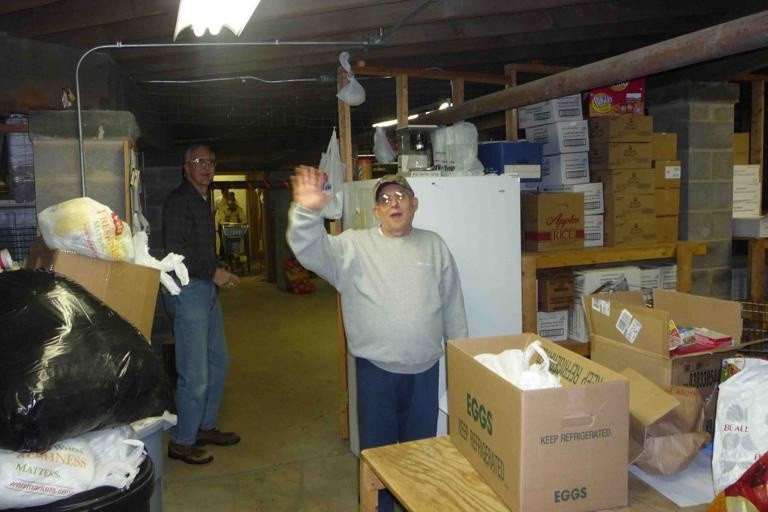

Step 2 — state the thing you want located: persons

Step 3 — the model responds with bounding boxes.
[160,143,241,464]
[215,188,247,260]
[285,164,470,512]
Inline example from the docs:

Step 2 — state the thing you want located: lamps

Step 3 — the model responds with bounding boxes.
[171,0,263,42]
[371,97,453,134]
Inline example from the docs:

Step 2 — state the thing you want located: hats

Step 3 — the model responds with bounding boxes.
[372,175,414,200]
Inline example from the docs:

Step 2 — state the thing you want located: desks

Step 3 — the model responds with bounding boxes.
[359,427,714,511]
[521,239,708,356]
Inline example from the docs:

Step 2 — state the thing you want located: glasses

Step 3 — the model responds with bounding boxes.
[377,190,411,205]
[187,159,216,168]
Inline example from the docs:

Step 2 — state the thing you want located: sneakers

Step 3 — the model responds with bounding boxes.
[197,430,240,446]
[168,443,214,464]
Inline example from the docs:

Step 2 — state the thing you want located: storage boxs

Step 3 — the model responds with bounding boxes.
[523,152,589,187]
[540,269,581,312]
[651,121,734,150]
[568,265,642,344]
[585,215,603,246]
[656,217,678,243]
[589,144,653,170]
[679,208,732,241]
[689,238,732,269]
[732,215,768,239]
[525,120,590,156]
[647,101,735,122]
[589,116,654,144]
[545,183,604,215]
[651,132,678,161]
[655,189,680,216]
[479,141,543,182]
[655,160,681,189]
[642,261,677,303]
[588,79,645,117]
[732,164,762,217]
[690,266,733,298]
[734,132,749,164]
[22,231,161,345]
[520,192,585,252]
[536,311,568,343]
[517,93,583,129]
[676,149,733,180]
[718,296,732,301]
[603,218,656,248]
[580,289,744,436]
[589,171,655,195]
[679,179,733,210]
[447,332,630,512]
[617,364,714,477]
[603,196,655,218]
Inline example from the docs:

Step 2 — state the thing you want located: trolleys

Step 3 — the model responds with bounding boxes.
[222,225,248,275]
